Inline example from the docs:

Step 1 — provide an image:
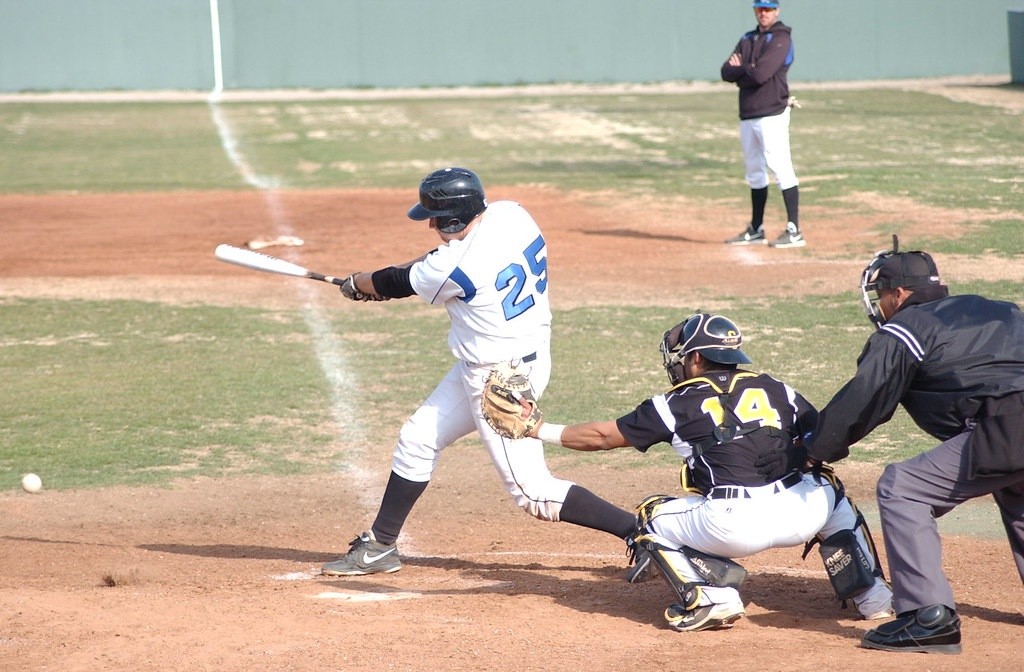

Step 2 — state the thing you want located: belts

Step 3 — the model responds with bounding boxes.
[522,352,537,363]
[711,469,805,499]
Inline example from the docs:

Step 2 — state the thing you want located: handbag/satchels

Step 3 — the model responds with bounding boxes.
[968,393,1024,482]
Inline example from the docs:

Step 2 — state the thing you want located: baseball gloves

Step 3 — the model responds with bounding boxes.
[480,362,544,441]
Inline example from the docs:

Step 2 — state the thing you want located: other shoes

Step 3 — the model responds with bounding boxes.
[842,592,893,620]
[663,603,743,632]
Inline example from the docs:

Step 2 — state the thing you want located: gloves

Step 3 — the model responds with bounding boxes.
[754,447,808,482]
[339,266,398,302]
[786,96,801,108]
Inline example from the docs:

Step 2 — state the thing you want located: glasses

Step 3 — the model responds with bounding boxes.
[756,7,773,12]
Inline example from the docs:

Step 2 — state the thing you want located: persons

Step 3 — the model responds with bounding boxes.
[720,0,806,248]
[322,169,659,585]
[480,314,893,632]
[752,234,1024,655]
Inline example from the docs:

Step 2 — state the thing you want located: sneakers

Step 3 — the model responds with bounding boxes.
[860,607,962,655]
[625,513,654,583]
[769,222,806,249]
[322,532,402,578]
[725,222,768,245]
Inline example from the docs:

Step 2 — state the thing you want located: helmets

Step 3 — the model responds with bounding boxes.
[407,167,488,233]
[679,314,753,364]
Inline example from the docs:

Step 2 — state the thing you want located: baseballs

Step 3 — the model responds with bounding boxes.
[21,473,42,493]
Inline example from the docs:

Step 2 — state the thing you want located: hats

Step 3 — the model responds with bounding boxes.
[753,0,779,9]
[865,251,940,291]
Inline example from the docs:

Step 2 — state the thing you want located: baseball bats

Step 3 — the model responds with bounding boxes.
[214,243,344,287]
